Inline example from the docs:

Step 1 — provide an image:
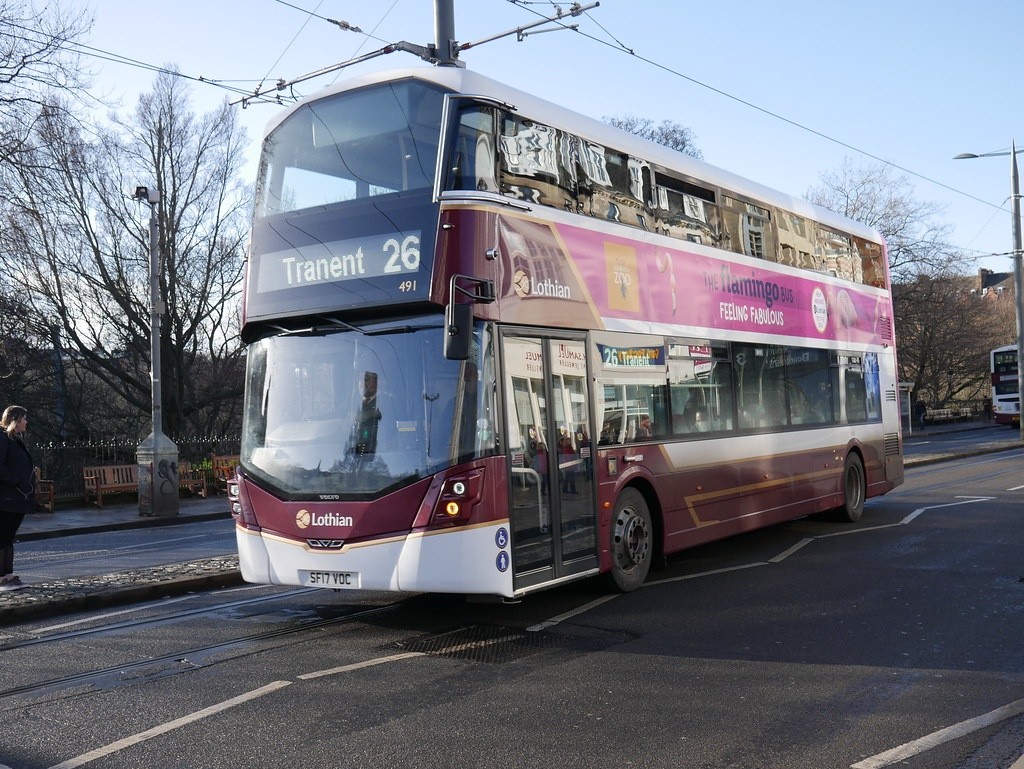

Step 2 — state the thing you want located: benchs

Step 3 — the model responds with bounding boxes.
[84,461,207,508]
[33,468,55,513]
[211,453,239,494]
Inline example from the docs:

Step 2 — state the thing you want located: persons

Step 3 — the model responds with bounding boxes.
[982,396,992,420]
[0,405,36,590]
[312,368,390,419]
[433,361,483,458]
[915,401,927,430]
[527,438,579,494]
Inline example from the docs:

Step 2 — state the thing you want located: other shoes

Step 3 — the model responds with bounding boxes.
[7,575,29,589]
[561,489,579,495]
[920,428,924,430]
[0,577,20,591]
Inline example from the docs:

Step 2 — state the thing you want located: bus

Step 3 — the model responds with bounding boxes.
[227,0,905,604]
[989,343,1020,428]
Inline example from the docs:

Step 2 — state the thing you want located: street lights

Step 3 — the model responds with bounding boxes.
[134,187,181,516]
[952,139,1024,441]
